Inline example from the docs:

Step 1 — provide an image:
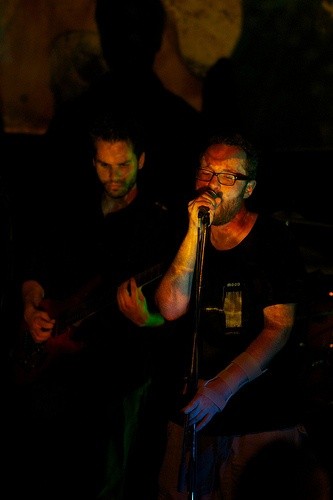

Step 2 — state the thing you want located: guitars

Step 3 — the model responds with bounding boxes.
[9,262,170,380]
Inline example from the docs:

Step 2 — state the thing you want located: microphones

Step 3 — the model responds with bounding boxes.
[197,189,218,218]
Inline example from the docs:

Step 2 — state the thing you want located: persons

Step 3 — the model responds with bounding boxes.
[152,133,301,500]
[18,117,188,500]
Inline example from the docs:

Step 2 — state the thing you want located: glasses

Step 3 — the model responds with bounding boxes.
[194,166,251,186]
